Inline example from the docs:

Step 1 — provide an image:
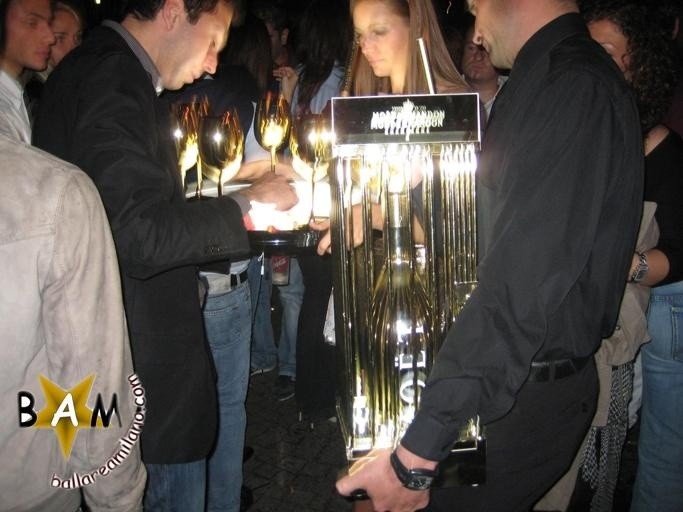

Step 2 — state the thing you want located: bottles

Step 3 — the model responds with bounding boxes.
[364,174,431,442]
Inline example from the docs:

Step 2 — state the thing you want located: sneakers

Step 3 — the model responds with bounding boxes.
[277,376,296,401]
[248,365,273,378]
[243,445,253,509]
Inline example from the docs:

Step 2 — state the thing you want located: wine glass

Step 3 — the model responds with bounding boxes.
[165,92,330,220]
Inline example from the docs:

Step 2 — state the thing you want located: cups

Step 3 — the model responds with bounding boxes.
[270,253,289,286]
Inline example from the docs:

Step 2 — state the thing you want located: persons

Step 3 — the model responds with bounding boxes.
[0,1,682,511]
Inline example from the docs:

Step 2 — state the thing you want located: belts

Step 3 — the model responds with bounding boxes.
[525,356,589,382]
[232,271,248,286]
